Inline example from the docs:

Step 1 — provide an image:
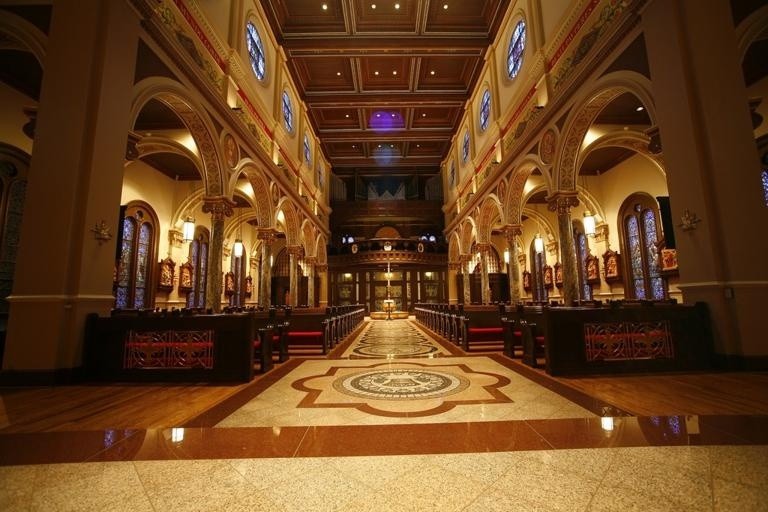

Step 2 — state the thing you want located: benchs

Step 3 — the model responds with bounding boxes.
[109,301,368,375]
[414,301,678,369]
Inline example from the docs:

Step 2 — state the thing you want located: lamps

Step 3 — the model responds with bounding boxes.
[183,180,195,243]
[580,168,595,237]
